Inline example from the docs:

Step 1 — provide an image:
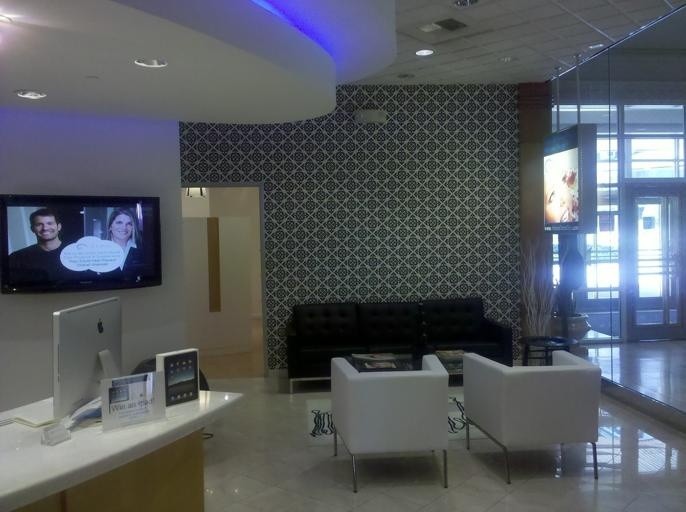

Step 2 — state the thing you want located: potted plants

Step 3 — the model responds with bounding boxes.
[550,277,589,340]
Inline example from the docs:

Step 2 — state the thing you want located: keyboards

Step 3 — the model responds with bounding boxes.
[12,396,62,428]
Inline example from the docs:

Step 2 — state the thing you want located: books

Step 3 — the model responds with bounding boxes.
[351,349,467,376]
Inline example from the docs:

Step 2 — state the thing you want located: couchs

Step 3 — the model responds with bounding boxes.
[285,296,515,396]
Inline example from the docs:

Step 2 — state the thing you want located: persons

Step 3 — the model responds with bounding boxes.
[9,207,73,273]
[86,207,143,279]
[545,159,574,223]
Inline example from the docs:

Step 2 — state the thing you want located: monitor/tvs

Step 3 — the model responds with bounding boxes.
[0,194,162,295]
[53,296,122,430]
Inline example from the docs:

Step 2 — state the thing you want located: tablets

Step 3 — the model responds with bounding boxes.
[165,351,198,407]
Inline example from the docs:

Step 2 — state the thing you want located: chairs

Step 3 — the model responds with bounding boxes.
[331,348,602,493]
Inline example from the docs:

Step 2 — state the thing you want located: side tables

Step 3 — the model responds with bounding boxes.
[519,336,578,365]
